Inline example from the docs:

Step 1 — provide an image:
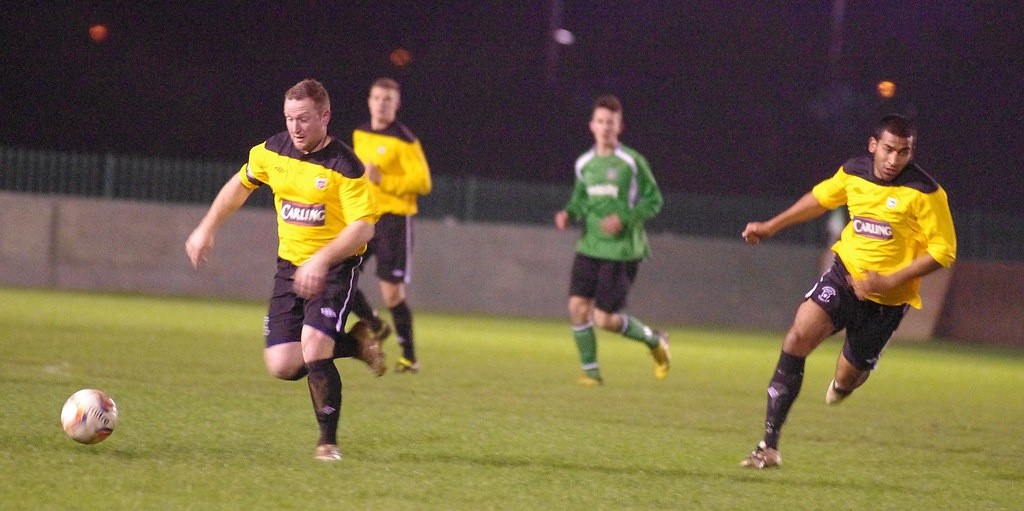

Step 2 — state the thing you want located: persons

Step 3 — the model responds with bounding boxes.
[352,79,432,372]
[186,80,387,461]
[554,95,671,386]
[739,116,958,471]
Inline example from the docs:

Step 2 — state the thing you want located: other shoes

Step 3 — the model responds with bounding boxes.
[372,324,392,342]
[576,376,605,388]
[348,317,387,376]
[741,441,781,471]
[394,356,419,374]
[312,444,341,463]
[825,378,851,406]
[649,330,670,379]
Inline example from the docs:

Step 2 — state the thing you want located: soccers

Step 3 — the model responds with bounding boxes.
[59,387,119,446]
